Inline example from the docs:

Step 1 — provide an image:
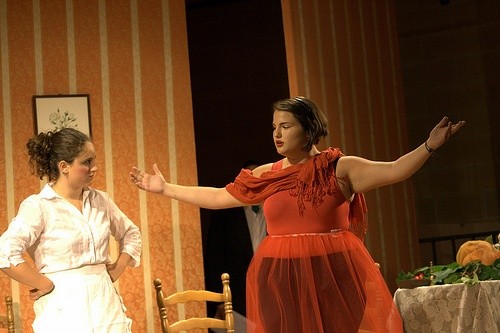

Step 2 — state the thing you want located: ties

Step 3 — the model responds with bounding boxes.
[251,205,260,215]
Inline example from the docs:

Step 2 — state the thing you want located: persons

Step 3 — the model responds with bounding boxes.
[0,127,143,333]
[130,96,464,333]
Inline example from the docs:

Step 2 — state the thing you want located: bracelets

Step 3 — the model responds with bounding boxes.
[424,142,441,159]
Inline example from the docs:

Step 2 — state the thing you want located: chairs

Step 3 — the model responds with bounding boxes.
[156,270,236,333]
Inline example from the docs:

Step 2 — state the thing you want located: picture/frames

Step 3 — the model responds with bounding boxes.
[31,94,93,141]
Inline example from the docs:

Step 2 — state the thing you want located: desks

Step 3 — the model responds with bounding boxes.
[390,276,500,333]
